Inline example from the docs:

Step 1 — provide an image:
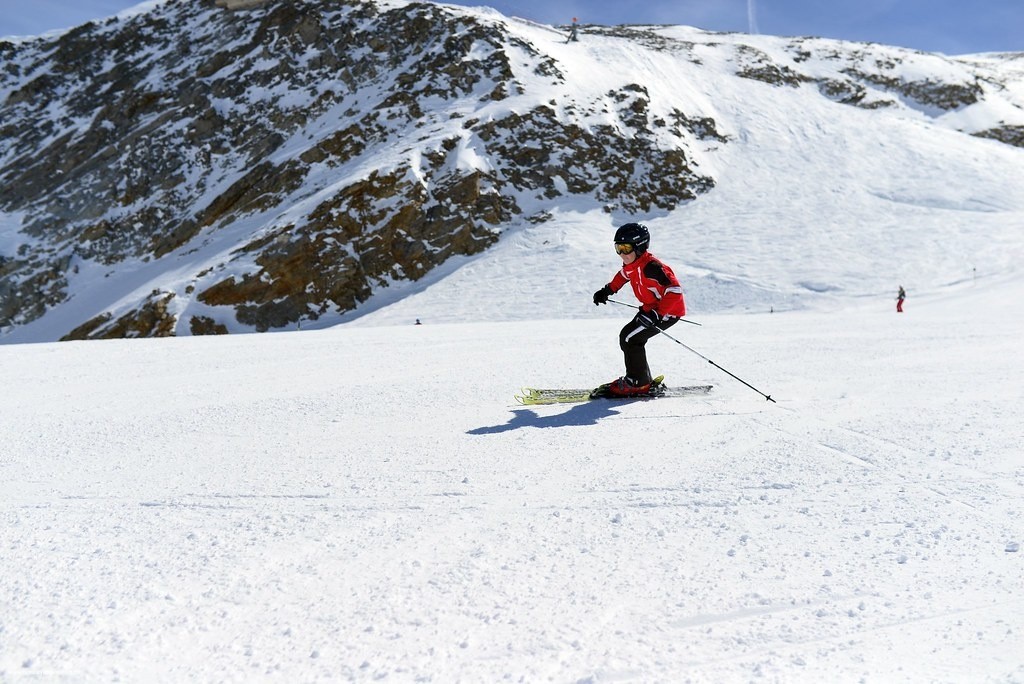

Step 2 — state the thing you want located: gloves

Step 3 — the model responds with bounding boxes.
[593,283,614,306]
[635,309,659,329]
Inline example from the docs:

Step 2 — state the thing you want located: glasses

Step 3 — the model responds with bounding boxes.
[614,241,636,255]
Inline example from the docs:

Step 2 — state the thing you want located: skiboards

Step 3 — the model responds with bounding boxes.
[516,383,715,405]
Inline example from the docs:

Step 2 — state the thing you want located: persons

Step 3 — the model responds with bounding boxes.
[896,286,906,313]
[593,223,685,395]
[571,17,580,42]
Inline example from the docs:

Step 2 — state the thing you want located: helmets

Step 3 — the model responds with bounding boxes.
[614,222,651,250]
